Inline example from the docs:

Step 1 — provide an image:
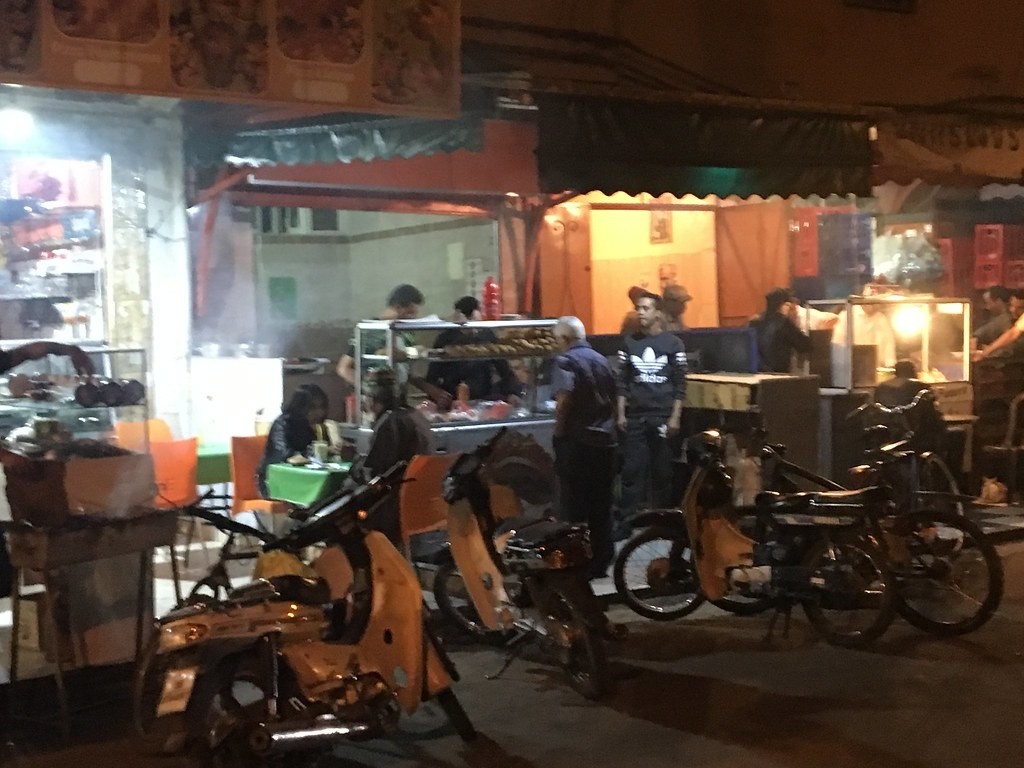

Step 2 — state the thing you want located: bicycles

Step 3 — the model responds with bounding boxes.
[607,386,1006,650]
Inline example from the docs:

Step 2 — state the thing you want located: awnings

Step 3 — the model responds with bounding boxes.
[533,92,874,199]
[226,94,494,170]
[877,109,1023,186]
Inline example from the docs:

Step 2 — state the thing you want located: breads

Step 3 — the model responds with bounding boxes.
[374,329,558,357]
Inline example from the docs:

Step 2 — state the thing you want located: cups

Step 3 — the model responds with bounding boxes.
[311,440,329,464]
[345,396,356,424]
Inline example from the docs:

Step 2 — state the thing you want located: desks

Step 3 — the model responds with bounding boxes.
[944,414,981,472]
[683,372,823,492]
[263,459,351,509]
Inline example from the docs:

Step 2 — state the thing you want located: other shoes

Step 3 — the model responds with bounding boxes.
[610,527,631,542]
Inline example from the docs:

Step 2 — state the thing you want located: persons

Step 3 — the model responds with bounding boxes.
[747,287,833,374]
[615,286,688,511]
[337,285,427,409]
[873,360,968,474]
[256,384,328,499]
[433,296,523,406]
[966,286,1024,451]
[662,286,692,332]
[550,317,620,577]
[336,369,435,547]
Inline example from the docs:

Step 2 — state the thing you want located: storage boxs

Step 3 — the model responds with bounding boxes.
[932,223,1024,298]
[16,591,53,650]
[51,546,154,668]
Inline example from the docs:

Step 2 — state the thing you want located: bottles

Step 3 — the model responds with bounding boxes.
[456,380,469,401]
[481,275,501,320]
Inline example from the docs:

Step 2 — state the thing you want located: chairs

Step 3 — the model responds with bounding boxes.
[984,394,1024,491]
[399,452,456,567]
[117,417,291,571]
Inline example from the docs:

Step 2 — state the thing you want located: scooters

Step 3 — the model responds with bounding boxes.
[433,424,629,702]
[129,456,479,768]
